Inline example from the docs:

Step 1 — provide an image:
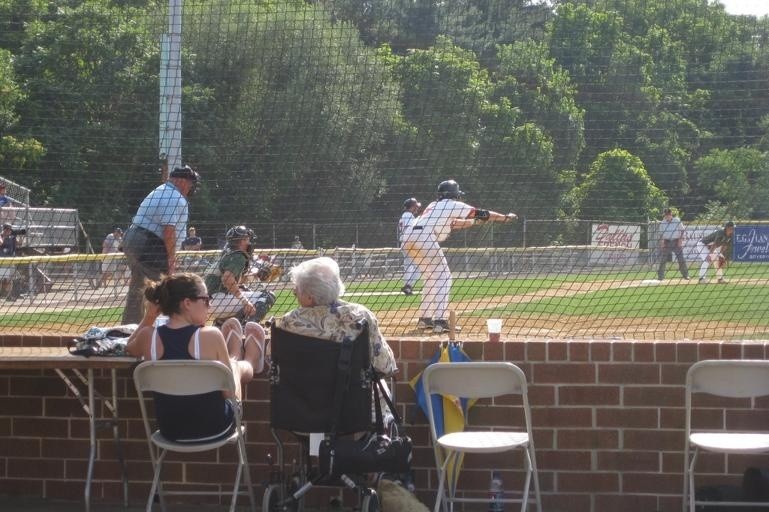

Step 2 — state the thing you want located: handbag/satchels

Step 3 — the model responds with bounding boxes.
[319,440,358,475]
[67,324,138,358]
[355,435,413,473]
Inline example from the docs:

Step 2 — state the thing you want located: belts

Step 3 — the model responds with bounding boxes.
[413,226,422,230]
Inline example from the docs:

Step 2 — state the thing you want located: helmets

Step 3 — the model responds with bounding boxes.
[170,166,201,196]
[403,197,422,209]
[226,226,258,256]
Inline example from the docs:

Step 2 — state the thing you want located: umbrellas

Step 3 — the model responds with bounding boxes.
[407,310,482,512]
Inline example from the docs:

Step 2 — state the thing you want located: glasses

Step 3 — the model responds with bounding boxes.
[195,297,210,306]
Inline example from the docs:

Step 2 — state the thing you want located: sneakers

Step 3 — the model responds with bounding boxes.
[717,279,727,283]
[698,279,709,283]
[418,317,434,328]
[432,320,461,333]
[401,285,412,295]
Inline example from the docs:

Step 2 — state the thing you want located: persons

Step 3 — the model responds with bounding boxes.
[696,221,736,284]
[124,271,268,446]
[265,254,414,492]
[119,165,202,327]
[396,197,422,296]
[655,207,691,281]
[402,178,519,335]
[1,182,305,328]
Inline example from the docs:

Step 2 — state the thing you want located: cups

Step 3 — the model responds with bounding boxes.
[487,319,503,342]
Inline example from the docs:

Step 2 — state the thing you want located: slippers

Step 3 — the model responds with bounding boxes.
[221,317,242,344]
[244,321,265,374]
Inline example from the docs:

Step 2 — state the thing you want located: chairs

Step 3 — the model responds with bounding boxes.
[131,360,257,512]
[683,358,769,511]
[422,361,543,512]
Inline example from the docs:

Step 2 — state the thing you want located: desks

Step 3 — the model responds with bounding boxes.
[1,347,146,512]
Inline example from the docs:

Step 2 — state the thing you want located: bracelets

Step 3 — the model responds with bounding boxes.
[503,213,510,223]
